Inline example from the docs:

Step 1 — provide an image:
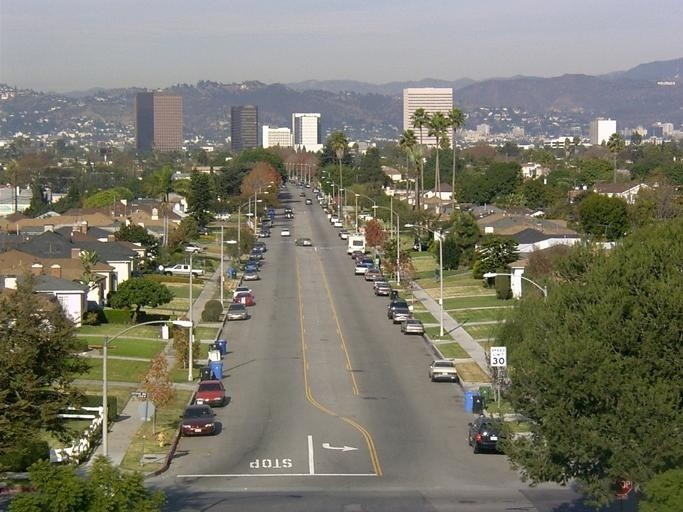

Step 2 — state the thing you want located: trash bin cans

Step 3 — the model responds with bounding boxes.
[473,396,484,413]
[228,268,237,280]
[464,392,479,411]
[209,344,219,351]
[210,361,223,380]
[215,340,227,355]
[201,368,211,382]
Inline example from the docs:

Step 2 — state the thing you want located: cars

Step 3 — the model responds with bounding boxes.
[351,250,424,336]
[243,241,265,281]
[232,286,251,300]
[194,381,225,407]
[179,404,216,436]
[427,360,457,382]
[235,293,254,306]
[294,238,312,245]
[258,179,350,240]
[226,303,248,320]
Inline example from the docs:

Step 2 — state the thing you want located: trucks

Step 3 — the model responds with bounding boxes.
[345,235,365,254]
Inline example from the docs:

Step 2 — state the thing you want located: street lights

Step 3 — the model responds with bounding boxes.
[320,170,377,237]
[482,272,548,303]
[404,223,443,338]
[370,205,401,288]
[220,213,253,310]
[188,239,237,383]
[238,180,276,244]
[101,319,192,461]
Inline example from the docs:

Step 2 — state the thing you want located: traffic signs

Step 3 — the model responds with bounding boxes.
[491,346,507,368]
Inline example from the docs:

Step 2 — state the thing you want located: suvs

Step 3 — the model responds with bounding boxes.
[466,417,510,456]
[162,264,204,279]
